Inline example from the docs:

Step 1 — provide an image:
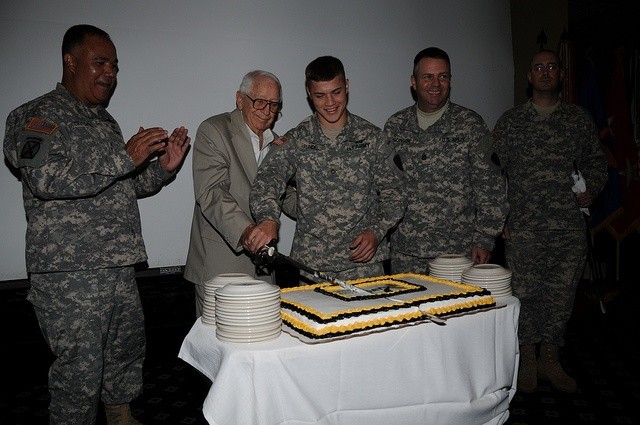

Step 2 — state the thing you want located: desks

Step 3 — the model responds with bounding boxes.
[178,300,521,425]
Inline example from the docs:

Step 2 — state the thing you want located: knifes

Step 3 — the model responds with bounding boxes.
[257,243,447,325]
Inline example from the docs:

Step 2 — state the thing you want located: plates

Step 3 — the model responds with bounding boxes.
[428,253,473,282]
[201,272,255,327]
[461,263,512,300]
[214,273,282,346]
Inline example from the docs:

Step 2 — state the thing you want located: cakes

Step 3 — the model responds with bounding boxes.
[277,273,496,340]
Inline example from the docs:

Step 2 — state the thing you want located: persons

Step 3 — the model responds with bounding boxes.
[183,70,298,320]
[383,46,511,274]
[491,45,608,394]
[248,56,407,285]
[3,24,190,424]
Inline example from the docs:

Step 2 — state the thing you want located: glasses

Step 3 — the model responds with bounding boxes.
[533,63,558,71]
[246,94,280,113]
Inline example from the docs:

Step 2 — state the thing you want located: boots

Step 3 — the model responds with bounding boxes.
[105,404,144,425]
[537,341,578,393]
[518,344,538,393]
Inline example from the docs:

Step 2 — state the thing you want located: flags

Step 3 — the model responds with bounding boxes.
[571,44,625,236]
[606,44,639,242]
